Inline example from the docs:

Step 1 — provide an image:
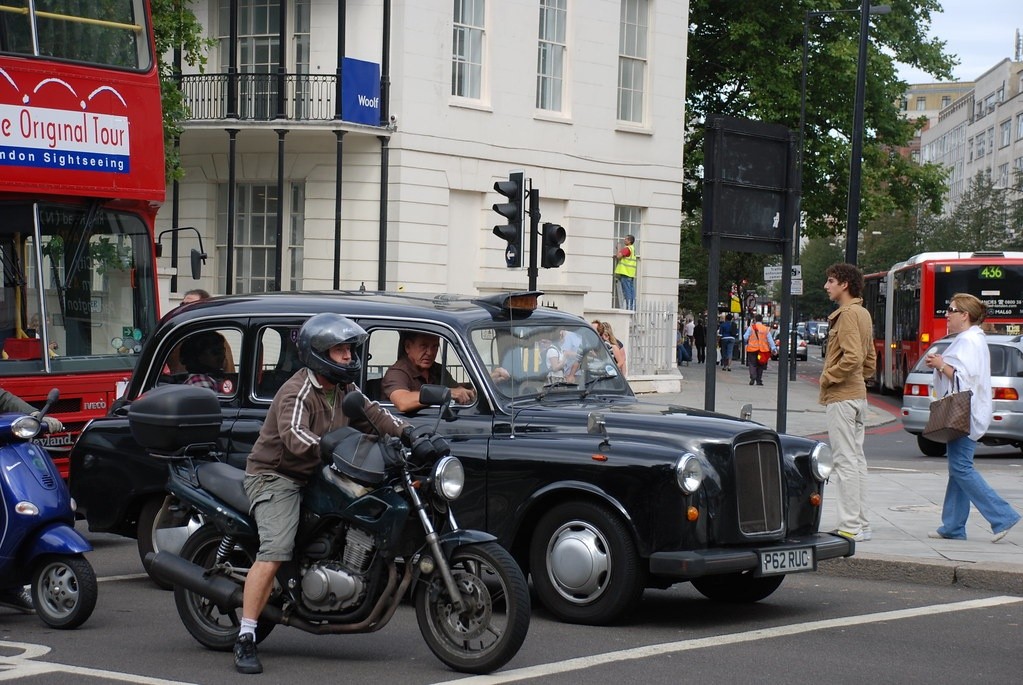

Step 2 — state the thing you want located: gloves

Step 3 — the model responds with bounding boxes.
[30,410,63,434]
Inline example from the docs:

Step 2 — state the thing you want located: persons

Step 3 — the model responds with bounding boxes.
[543,315,626,387]
[379,327,510,414]
[743,313,778,386]
[614,234,637,311]
[719,313,737,371]
[30,312,58,351]
[676,315,707,367]
[232,312,417,674]
[925,293,1021,544]
[820,264,876,542]
[169,288,237,374]
[0,387,63,434]
[178,330,227,393]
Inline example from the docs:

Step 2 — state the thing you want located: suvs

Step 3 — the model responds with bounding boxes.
[66,288,858,628]
[898,330,1023,459]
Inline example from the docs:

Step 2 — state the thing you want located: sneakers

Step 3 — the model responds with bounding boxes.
[0,590,37,614]
[233,633,262,673]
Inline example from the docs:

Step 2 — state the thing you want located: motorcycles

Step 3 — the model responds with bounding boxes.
[125,381,533,676]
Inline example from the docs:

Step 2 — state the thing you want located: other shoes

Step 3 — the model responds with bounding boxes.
[722,368,726,371]
[728,368,731,371]
[757,381,763,385]
[829,529,863,541]
[750,377,755,385]
[928,530,944,539]
[991,525,1014,543]
[863,532,871,539]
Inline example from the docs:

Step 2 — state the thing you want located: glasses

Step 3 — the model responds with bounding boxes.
[947,307,962,313]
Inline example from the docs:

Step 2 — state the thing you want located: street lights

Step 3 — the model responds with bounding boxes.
[790,4,895,384]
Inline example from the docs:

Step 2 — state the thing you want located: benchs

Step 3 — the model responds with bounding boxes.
[169,369,295,400]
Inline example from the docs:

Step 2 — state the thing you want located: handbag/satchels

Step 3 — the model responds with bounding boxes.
[757,352,770,365]
[922,369,973,443]
[538,347,559,378]
[716,346,722,362]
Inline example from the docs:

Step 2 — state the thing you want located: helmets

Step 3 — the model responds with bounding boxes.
[298,311,362,383]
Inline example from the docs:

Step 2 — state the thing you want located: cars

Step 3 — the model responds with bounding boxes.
[767,318,830,361]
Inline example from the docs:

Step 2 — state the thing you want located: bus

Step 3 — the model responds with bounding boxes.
[859,249,1023,400]
[0,0,212,479]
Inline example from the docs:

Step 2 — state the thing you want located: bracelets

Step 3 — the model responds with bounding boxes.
[940,363,948,373]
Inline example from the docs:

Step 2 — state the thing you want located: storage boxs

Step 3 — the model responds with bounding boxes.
[129,385,223,457]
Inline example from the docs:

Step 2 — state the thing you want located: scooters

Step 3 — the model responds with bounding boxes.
[0,387,99,631]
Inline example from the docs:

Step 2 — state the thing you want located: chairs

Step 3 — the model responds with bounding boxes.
[365,378,384,402]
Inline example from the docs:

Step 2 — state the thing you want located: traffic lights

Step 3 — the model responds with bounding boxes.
[540,223,567,269]
[492,169,526,268]
